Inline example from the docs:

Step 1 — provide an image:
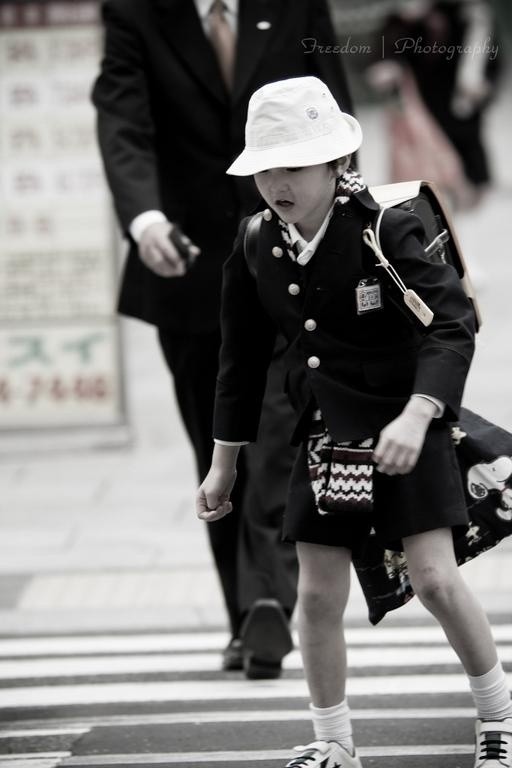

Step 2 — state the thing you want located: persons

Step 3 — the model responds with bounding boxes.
[191,75,511,767]
[86,1,360,680]
[370,0,502,213]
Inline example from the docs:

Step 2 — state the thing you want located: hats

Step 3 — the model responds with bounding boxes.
[226,76,363,177]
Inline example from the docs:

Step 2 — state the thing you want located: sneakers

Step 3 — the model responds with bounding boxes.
[283,739,363,768]
[473,690,511,768]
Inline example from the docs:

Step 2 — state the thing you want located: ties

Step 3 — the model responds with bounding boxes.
[208,2,236,98]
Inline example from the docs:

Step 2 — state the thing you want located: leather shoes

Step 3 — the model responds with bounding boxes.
[240,599,294,679]
[222,638,242,671]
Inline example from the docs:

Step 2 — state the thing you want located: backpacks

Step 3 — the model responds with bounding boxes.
[245,180,483,338]
